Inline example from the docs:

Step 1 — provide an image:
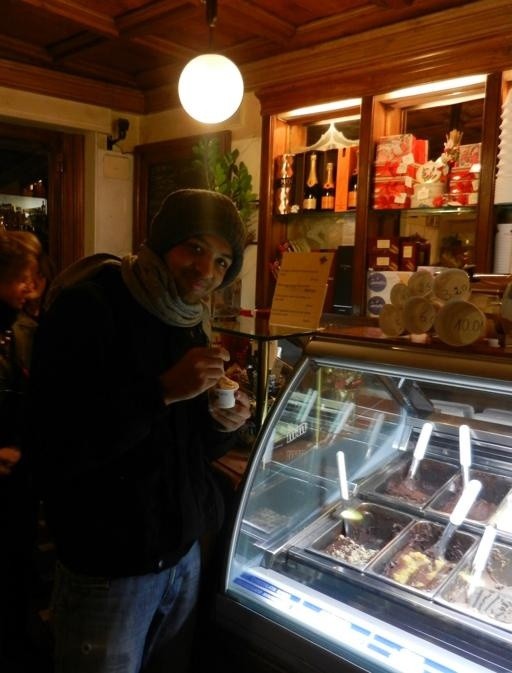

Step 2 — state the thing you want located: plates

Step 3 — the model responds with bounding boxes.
[415,160,441,183]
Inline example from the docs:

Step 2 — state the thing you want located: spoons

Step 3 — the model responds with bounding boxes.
[221,370,236,388]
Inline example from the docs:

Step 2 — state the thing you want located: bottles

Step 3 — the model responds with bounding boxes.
[493,222,511,274]
[0,200,49,258]
[303,154,320,214]
[268,367,277,388]
[321,161,335,212]
[347,148,360,209]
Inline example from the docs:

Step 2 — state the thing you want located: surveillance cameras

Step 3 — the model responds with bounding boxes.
[119,119,129,130]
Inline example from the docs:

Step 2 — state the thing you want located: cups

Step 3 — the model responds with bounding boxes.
[208,379,240,409]
[378,267,495,347]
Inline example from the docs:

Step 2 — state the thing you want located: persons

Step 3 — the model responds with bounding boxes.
[35,188,252,672]
[14,256,59,371]
[0,229,42,478]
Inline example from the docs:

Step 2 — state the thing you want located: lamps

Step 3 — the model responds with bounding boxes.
[177,0,245,126]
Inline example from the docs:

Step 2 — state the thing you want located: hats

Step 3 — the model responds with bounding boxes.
[147,188,246,293]
